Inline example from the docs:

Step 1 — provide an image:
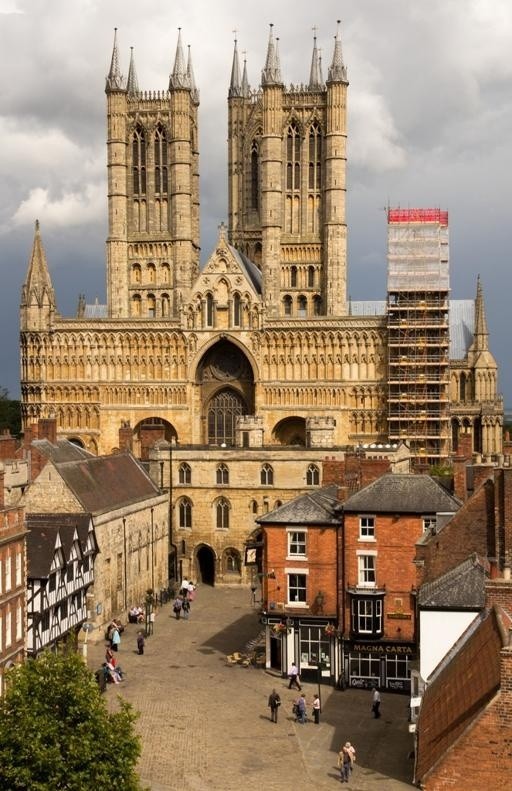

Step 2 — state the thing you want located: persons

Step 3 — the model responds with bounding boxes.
[137,632,144,655]
[337,747,354,783]
[313,694,320,724]
[345,741,356,771]
[335,669,346,691]
[288,662,302,691]
[295,693,306,724]
[162,578,196,619]
[129,605,155,638]
[267,688,281,723]
[95,619,123,684]
[372,687,381,719]
